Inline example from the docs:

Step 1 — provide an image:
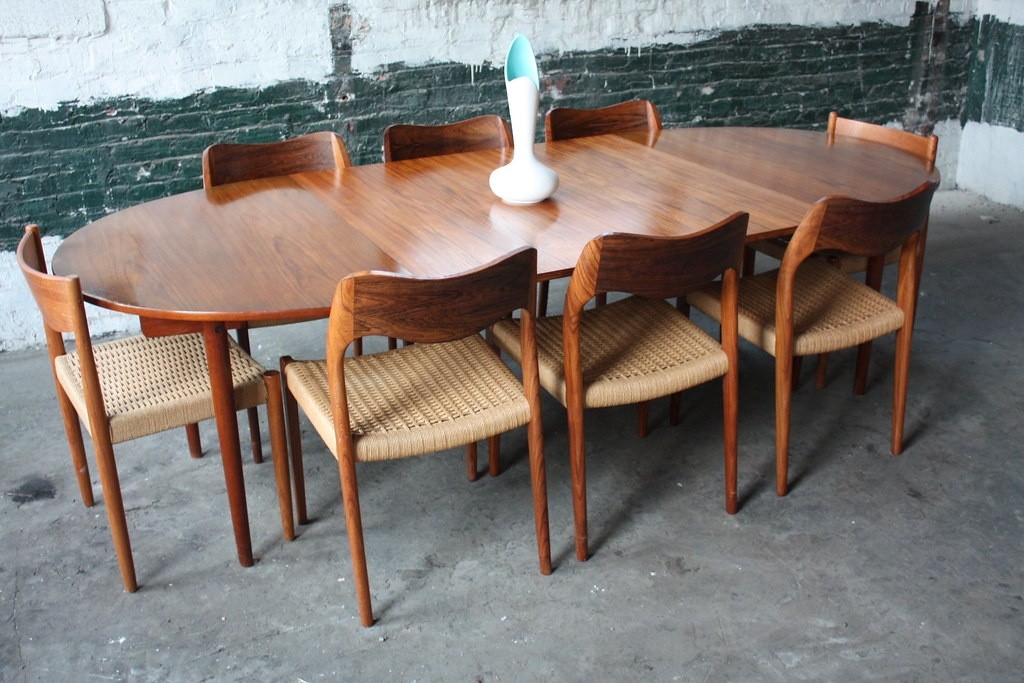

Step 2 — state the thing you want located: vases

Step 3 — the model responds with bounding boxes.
[488,35,560,207]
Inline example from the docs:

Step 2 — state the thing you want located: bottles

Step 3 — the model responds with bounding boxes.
[489,35,559,206]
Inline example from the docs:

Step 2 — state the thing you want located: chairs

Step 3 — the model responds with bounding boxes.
[17,225,296,594]
[381,118,513,352]
[741,111,938,400]
[535,100,666,317]
[668,179,940,498]
[484,210,749,563]
[200,132,364,464]
[280,244,552,629]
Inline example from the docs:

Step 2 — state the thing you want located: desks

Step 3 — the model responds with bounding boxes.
[50,126,941,569]
